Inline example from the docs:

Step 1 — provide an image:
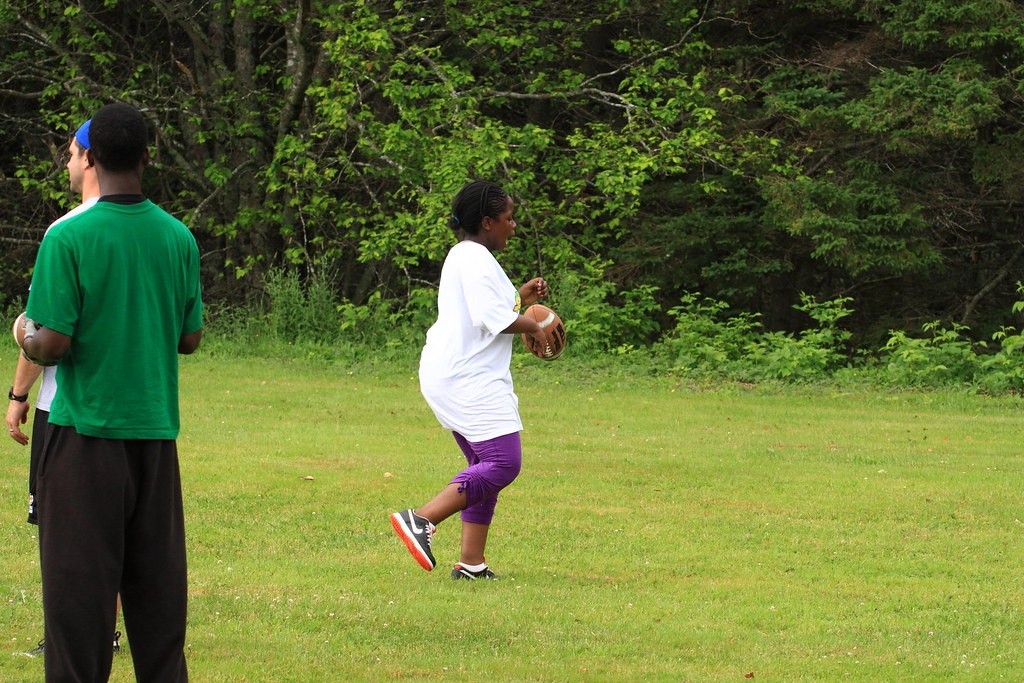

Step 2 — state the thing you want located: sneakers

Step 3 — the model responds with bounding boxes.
[451,565,500,582]
[15,641,46,661]
[390,508,436,571]
[113,632,121,652]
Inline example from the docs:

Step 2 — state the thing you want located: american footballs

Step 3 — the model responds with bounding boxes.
[12,311,30,347]
[521,304,566,361]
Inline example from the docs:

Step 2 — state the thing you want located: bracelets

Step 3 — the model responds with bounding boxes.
[8,386,29,403]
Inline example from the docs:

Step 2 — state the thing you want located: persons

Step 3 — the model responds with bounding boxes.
[19,104,204,683]
[389,179,551,581]
[6,121,121,659]
